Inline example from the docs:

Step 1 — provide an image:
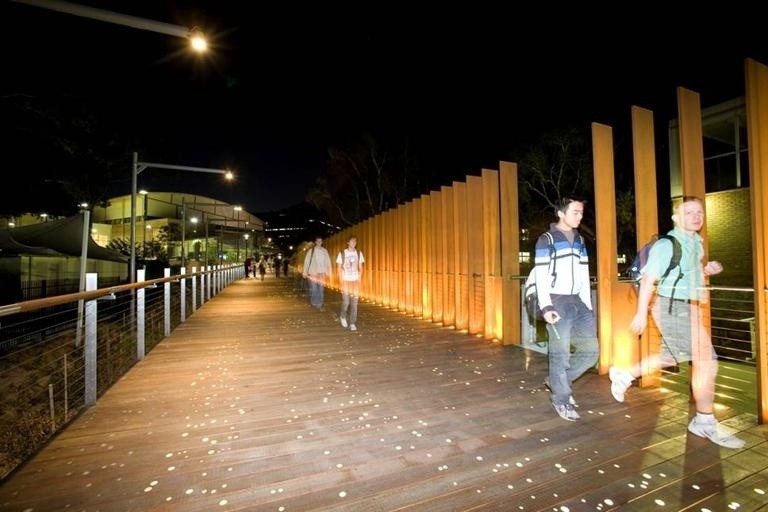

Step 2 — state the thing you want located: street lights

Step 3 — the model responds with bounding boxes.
[125,147,240,341]
[1,2,218,57]
[176,195,297,282]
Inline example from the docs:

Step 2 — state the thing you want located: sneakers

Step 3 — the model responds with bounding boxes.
[350,323,358,331]
[607,364,635,404]
[552,397,583,422]
[687,416,747,449]
[339,314,348,328]
[544,375,578,407]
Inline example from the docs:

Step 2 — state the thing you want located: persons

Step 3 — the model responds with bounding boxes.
[244,253,290,282]
[301,238,332,309]
[534,195,599,421]
[336,234,365,330]
[609,197,744,447]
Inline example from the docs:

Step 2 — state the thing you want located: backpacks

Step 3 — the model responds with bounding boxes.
[523,230,563,324]
[626,234,683,301]
